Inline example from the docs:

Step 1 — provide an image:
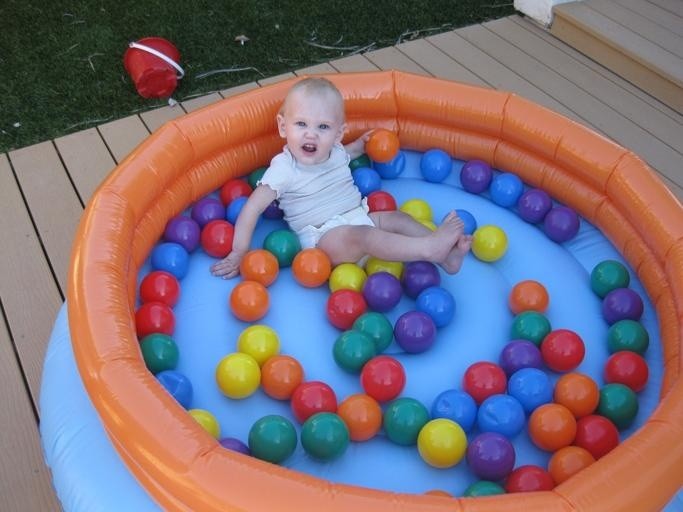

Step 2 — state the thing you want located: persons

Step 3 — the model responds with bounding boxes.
[210,78,474,278]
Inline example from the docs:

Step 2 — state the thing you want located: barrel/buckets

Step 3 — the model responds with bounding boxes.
[123,37,185,99]
